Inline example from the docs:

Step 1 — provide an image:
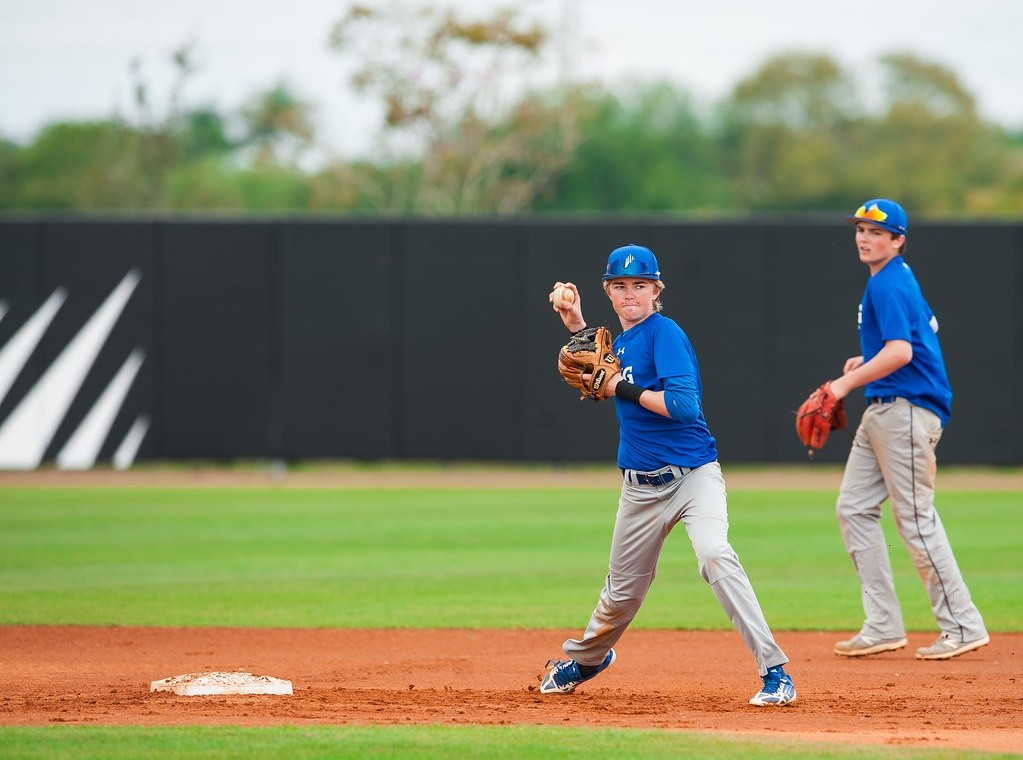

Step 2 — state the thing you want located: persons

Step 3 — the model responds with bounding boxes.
[795,199,992,660]
[538,242,799,709]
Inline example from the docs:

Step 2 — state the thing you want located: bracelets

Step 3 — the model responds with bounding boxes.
[615,379,647,406]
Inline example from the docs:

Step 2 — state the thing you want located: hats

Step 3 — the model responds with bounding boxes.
[846,199,908,235]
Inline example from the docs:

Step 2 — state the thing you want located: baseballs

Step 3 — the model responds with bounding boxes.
[552,285,575,309]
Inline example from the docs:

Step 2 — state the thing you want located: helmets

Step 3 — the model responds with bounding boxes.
[602,243,660,280]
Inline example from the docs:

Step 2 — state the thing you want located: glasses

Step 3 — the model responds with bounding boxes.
[854,206,907,233]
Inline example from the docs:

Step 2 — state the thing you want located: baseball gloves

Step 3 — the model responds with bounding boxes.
[796,380,848,448]
[556,325,622,403]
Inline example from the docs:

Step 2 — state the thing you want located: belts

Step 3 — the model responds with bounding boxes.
[622,467,694,486]
[873,396,896,402]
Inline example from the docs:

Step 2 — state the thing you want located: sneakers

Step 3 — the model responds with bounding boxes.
[748,665,796,706]
[540,648,617,694]
[834,633,908,657]
[916,633,991,659]
